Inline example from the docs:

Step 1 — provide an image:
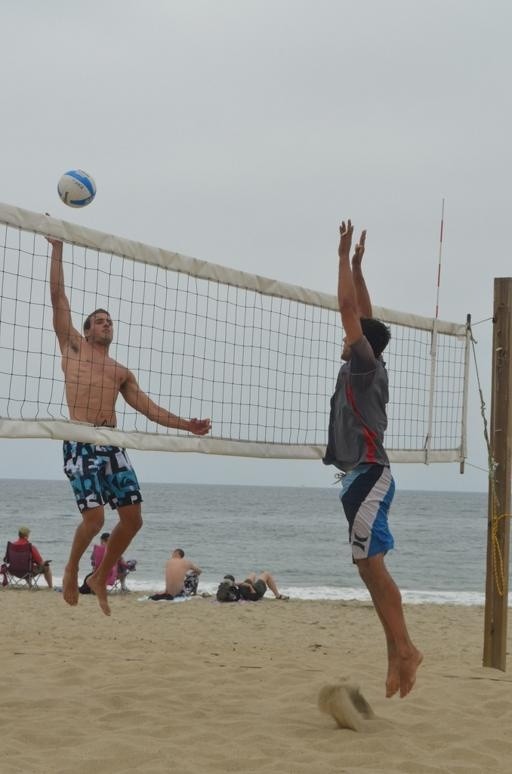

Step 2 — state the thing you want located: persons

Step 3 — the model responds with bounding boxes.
[320,214,426,700]
[163,547,203,600]
[40,211,215,616]
[4,525,54,588]
[220,571,290,602]
[89,531,132,594]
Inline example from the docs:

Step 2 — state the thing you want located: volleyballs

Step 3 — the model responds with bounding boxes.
[57,170,97,208]
